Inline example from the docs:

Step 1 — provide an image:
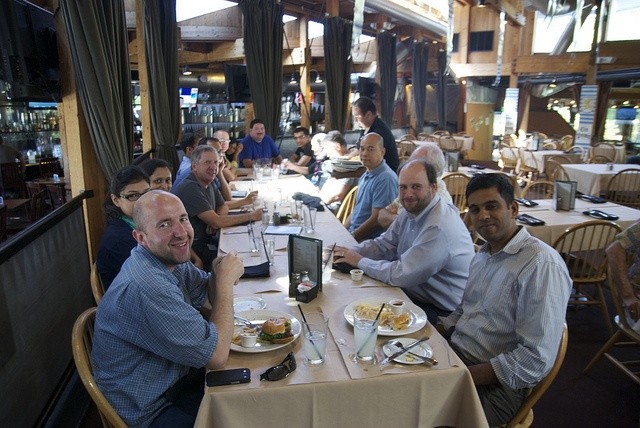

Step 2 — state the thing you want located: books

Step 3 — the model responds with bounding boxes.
[552,181,577,212]
[445,152,459,172]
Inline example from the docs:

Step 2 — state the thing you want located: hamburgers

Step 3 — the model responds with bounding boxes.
[258,317,294,344]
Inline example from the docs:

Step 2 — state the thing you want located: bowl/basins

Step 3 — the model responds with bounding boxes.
[239,332,258,348]
[350,268,364,281]
[388,299,406,314]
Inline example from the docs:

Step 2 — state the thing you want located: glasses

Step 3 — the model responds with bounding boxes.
[295,135,306,140]
[118,193,143,201]
[260,352,296,381]
[219,138,231,142]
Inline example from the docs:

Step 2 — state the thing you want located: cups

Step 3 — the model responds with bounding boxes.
[301,312,330,368]
[0,196,4,205]
[271,168,280,190]
[259,235,275,267]
[290,200,303,224]
[280,193,288,207]
[269,200,276,223]
[254,164,263,183]
[321,247,334,283]
[53,174,58,182]
[247,223,262,252]
[302,207,317,235]
[354,314,380,364]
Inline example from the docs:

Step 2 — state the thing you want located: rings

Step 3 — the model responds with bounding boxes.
[342,251,344,257]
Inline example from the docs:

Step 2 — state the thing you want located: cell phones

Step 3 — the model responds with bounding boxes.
[205,368,251,387]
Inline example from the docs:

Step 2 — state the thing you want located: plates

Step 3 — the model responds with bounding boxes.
[344,296,428,337]
[382,338,433,365]
[233,295,267,312]
[230,309,301,354]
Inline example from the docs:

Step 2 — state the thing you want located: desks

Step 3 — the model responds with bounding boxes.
[3,197,31,229]
[25,177,71,184]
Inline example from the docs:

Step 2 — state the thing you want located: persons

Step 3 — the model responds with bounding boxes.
[328,161,475,323]
[199,130,242,180]
[140,159,204,273]
[176,133,202,178]
[604,219,639,333]
[90,189,244,428]
[330,97,399,179]
[0,134,25,176]
[435,172,573,427]
[344,133,398,244]
[238,119,281,169]
[378,141,453,229]
[95,165,153,295]
[168,138,257,209]
[169,145,262,275]
[284,127,360,213]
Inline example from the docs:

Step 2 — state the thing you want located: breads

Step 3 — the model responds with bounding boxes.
[393,313,410,331]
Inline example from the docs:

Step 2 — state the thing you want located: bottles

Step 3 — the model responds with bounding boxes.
[228,108,234,122]
[289,272,301,298]
[201,126,207,138]
[207,108,213,123]
[272,211,280,225]
[261,208,270,225]
[301,270,310,284]
[207,125,214,139]
[234,125,239,140]
[229,124,234,141]
[200,106,207,123]
[234,108,238,122]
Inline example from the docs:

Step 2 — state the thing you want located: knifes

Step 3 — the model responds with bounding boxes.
[379,336,430,366]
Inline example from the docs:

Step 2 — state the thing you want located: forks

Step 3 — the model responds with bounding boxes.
[384,339,438,366]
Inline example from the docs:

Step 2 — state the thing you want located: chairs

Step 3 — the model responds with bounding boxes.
[0,162,26,198]
[541,142,557,150]
[551,220,623,336]
[45,183,65,210]
[336,186,359,224]
[5,188,46,235]
[503,320,569,427]
[441,173,472,214]
[432,130,450,136]
[591,142,617,162]
[546,154,572,174]
[581,263,640,383]
[417,133,428,137]
[438,134,457,151]
[566,145,585,163]
[520,180,555,199]
[605,168,640,210]
[399,134,417,140]
[71,306,133,428]
[589,154,613,163]
[397,139,417,155]
[90,261,108,305]
[398,155,410,161]
[39,156,60,177]
[511,146,539,184]
[423,136,439,145]
[452,131,466,136]
[498,143,518,173]
[559,135,573,151]
[26,182,40,195]
[547,159,570,198]
[529,133,548,140]
